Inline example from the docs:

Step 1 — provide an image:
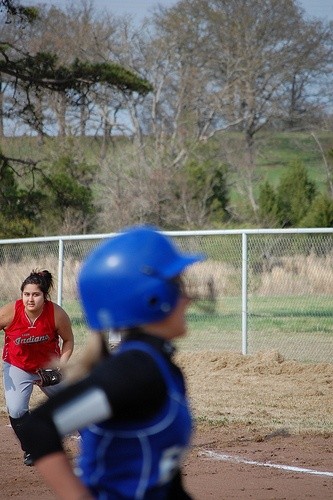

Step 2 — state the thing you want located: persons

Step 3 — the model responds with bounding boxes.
[19,226,206,500]
[0,266,74,466]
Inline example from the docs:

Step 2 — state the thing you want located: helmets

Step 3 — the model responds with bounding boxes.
[76,224,208,328]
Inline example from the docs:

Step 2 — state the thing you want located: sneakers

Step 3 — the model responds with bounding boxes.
[24,451,33,466]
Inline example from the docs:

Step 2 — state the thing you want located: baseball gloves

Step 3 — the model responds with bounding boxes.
[33,366,64,387]
